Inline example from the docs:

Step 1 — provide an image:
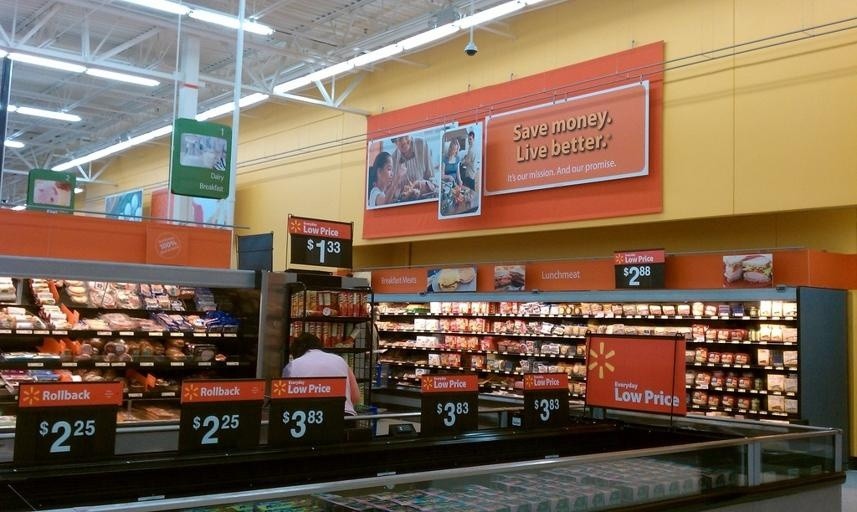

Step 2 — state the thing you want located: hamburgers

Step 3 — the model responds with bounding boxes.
[439,271,457,291]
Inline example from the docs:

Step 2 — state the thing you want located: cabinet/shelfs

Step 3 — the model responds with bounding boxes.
[281,278,376,407]
[375,285,850,465]
[1,278,254,456]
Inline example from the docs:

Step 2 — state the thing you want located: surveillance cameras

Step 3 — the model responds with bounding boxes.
[464,41,478,56]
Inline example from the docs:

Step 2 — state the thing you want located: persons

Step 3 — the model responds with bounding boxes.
[368,128,479,215]
[279,330,361,442]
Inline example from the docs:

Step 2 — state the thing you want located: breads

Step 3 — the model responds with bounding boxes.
[459,269,475,283]
